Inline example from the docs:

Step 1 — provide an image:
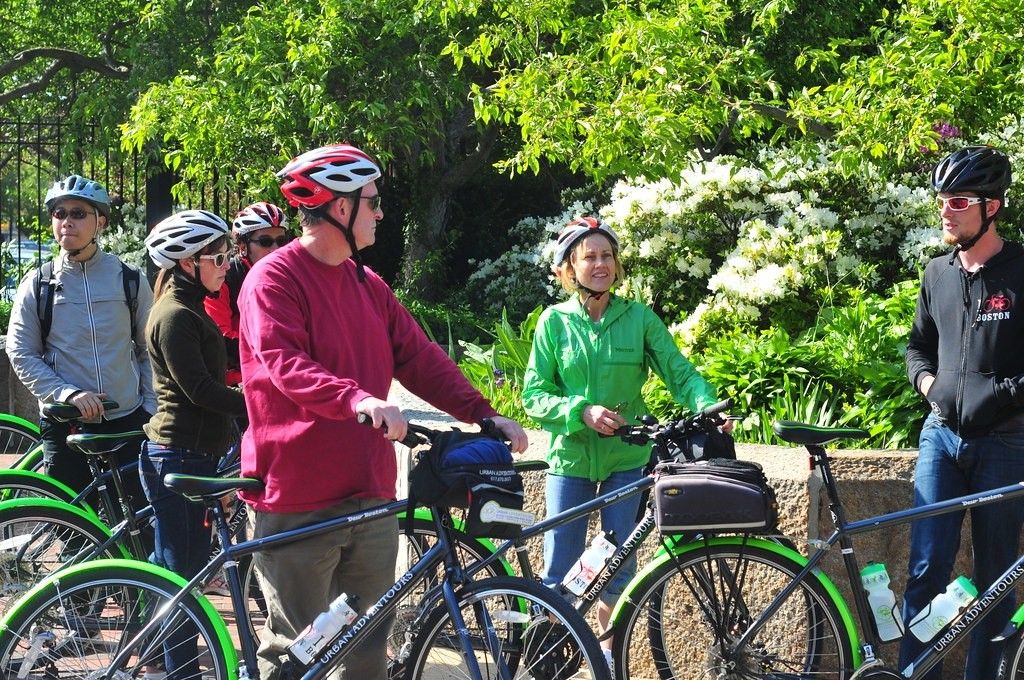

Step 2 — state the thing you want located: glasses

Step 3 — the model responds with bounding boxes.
[52,206,95,220]
[332,195,382,213]
[936,194,992,212]
[247,236,288,248]
[189,250,232,268]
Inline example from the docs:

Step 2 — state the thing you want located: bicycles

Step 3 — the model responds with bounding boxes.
[647,419,1024,679]
[0,398,827,680]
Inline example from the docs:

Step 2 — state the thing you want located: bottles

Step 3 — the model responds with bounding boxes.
[908,575,979,644]
[858,558,905,642]
[285,592,360,671]
[562,530,619,598]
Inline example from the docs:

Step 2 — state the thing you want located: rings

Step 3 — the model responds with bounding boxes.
[601,425,604,430]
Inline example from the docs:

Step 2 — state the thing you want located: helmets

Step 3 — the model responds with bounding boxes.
[553,217,619,268]
[231,202,289,244]
[930,144,1012,194]
[44,174,111,218]
[276,144,382,210]
[143,209,228,270]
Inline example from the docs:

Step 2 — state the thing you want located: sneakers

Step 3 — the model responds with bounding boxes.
[201,570,232,598]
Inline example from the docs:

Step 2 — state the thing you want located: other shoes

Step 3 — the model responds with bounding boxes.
[141,670,168,680]
[71,630,101,655]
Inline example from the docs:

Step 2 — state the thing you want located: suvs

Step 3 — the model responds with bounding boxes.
[4,240,53,302]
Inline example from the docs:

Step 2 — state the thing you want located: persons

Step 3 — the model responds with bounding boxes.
[898,146,1024,680]
[522,216,733,668]
[139,210,246,680]
[5,174,161,651]
[204,201,289,596]
[235,144,528,680]
